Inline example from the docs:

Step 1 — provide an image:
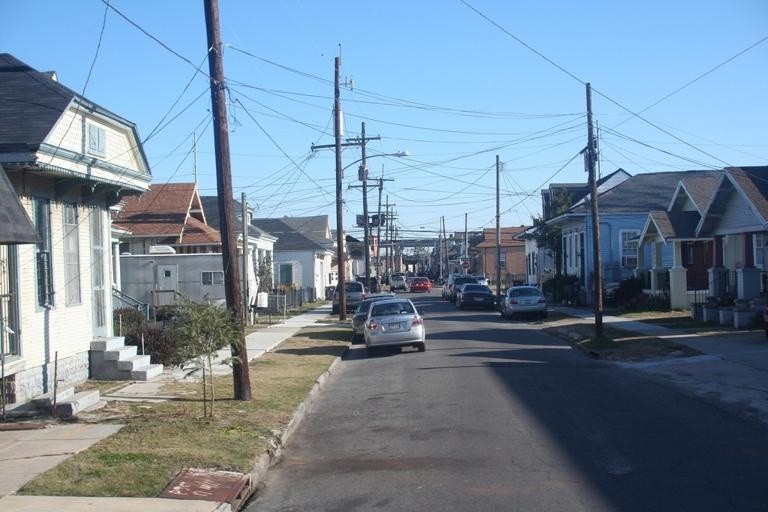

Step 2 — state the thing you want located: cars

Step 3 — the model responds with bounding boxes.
[500,285,548,321]
[442,273,496,311]
[332,271,433,356]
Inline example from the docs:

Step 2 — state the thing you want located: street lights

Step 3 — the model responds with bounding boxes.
[419,227,442,283]
[335,150,410,320]
